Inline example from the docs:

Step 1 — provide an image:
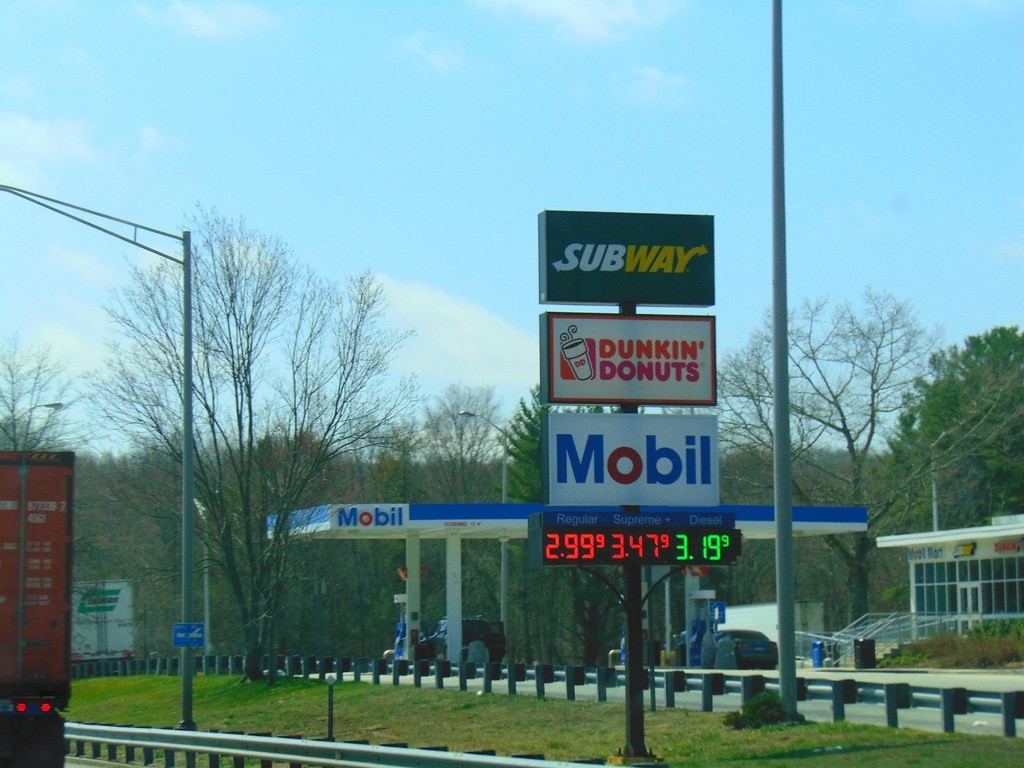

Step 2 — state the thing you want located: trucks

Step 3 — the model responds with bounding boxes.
[1,448,80,768]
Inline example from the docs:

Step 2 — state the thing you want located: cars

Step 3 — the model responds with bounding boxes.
[714,628,778,671]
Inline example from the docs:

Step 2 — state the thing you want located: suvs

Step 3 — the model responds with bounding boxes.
[417,615,507,666]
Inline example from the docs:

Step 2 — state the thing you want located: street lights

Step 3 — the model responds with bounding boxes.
[461,409,510,637]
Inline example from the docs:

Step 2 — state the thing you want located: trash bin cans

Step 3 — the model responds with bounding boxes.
[827,643,840,668]
[812,641,825,668]
[854,638,875,669]
